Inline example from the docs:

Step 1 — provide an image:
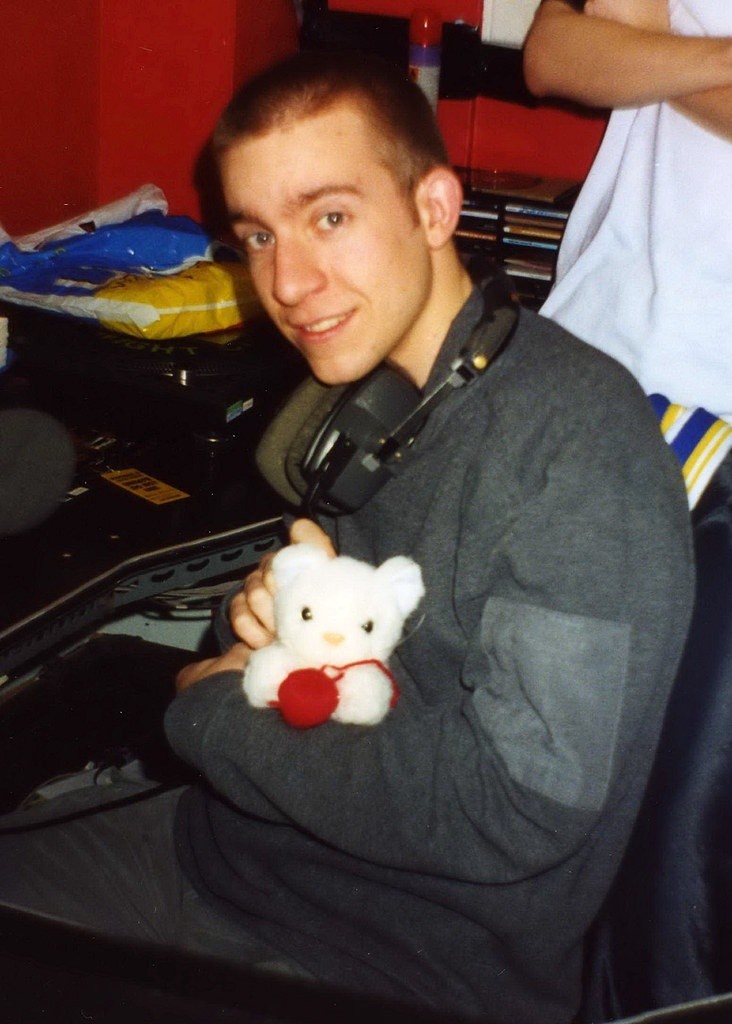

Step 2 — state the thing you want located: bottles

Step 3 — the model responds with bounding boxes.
[406,7,438,109]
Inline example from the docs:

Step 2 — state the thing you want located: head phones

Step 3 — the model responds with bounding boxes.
[256,269,521,518]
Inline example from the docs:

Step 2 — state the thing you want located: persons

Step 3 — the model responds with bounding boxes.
[0,47,694,1022]
[523,1,730,1023]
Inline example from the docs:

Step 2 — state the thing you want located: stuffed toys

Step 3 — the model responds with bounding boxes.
[243,543,426,728]
